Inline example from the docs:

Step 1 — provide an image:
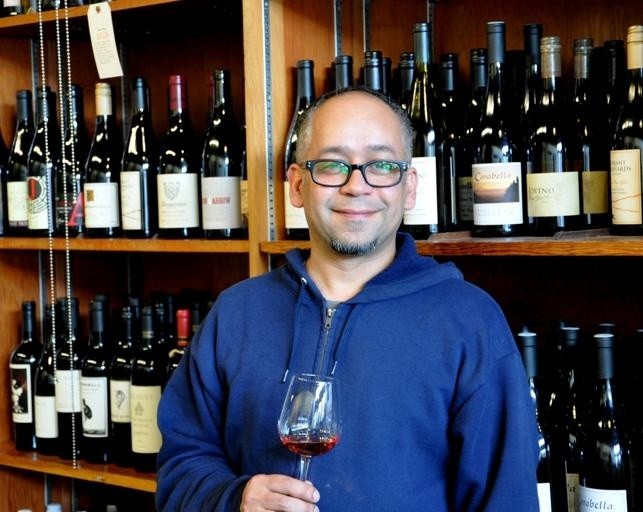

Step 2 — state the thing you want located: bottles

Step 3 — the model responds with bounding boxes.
[7,287,221,474]
[0,65,244,239]
[281,20,642,241]
[514,311,642,512]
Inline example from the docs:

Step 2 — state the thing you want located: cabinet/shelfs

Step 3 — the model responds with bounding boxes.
[0,0,254,512]
[254,1,643,512]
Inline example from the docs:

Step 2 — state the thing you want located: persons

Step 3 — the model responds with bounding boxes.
[154,85,540,512]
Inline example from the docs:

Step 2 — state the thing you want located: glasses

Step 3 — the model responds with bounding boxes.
[298,159,408,189]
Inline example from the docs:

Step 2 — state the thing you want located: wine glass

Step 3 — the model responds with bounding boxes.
[274,371,339,482]
